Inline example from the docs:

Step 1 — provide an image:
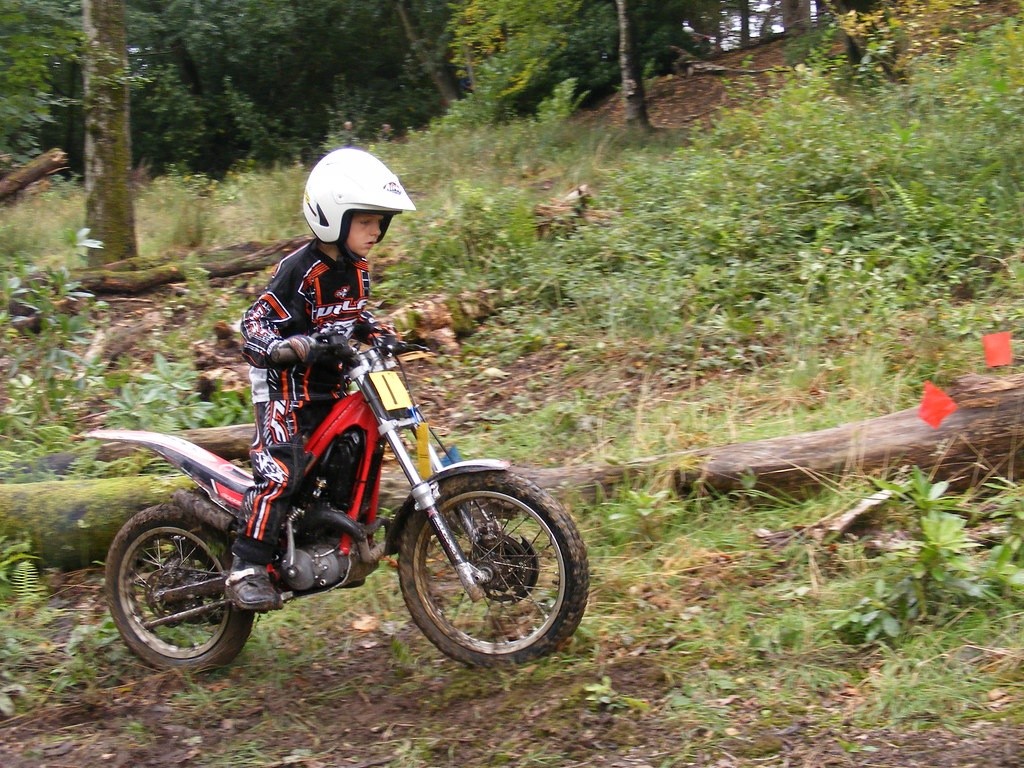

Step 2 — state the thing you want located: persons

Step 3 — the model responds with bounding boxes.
[225,148,416,614]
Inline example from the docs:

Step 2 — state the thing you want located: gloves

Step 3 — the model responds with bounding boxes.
[369,324,403,358]
[269,333,318,364]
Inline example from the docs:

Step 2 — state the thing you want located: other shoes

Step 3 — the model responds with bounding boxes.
[225,567,285,612]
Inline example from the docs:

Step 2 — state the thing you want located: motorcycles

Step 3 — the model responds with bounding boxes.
[81,320,586,679]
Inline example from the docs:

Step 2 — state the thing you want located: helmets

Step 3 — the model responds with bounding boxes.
[302,148,417,245]
